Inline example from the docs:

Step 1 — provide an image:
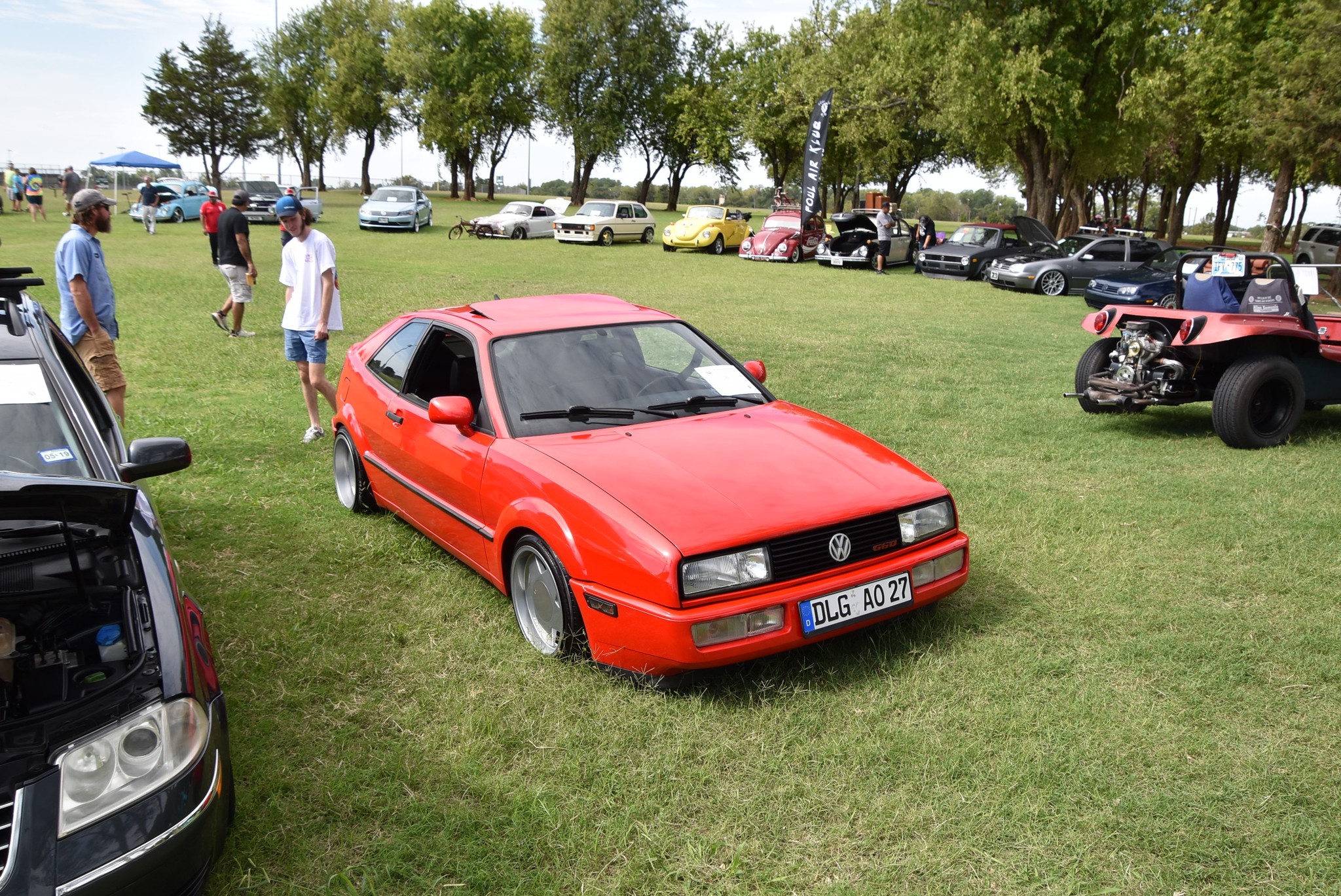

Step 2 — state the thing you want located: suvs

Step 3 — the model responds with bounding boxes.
[1062,250,1341,448]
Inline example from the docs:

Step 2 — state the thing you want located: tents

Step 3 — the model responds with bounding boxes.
[85,149,182,215]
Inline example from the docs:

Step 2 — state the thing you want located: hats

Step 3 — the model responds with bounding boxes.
[65,165,73,169]
[1095,214,1103,219]
[232,189,253,203]
[284,188,294,196]
[275,195,303,221]
[882,202,893,207]
[208,191,219,198]
[1124,216,1130,219]
[72,188,117,212]
[920,215,929,227]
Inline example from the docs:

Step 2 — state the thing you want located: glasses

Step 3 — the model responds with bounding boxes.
[93,204,109,211]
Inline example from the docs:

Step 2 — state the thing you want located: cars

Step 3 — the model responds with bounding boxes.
[739,187,834,264]
[917,223,1023,281]
[1084,245,1274,312]
[662,204,756,256]
[332,293,970,677]
[475,197,573,240]
[1291,223,1341,276]
[814,208,921,271]
[985,215,1180,297]
[235,180,323,225]
[0,267,238,896]
[128,177,224,224]
[559,200,657,247]
[359,185,433,233]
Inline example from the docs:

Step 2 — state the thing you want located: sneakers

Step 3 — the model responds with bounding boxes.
[876,269,889,275]
[211,310,230,333]
[300,426,324,444]
[229,328,256,338]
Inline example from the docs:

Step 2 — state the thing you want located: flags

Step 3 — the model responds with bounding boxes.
[800,88,835,227]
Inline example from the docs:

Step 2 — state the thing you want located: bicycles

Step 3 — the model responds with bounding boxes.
[448,215,495,241]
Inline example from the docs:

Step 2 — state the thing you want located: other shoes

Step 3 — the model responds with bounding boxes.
[150,232,155,234]
[12,207,30,212]
[62,212,71,217]
[146,226,150,232]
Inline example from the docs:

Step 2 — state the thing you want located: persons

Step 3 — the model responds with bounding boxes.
[874,202,896,275]
[1076,220,1097,234]
[22,167,48,223]
[135,175,159,235]
[58,168,75,215]
[1092,214,1104,234]
[12,168,23,211]
[274,194,345,444]
[1095,220,1111,234]
[3,162,16,210]
[278,188,301,247]
[209,189,259,338]
[1113,215,1131,236]
[199,188,230,267]
[910,214,937,273]
[62,165,82,216]
[53,189,126,429]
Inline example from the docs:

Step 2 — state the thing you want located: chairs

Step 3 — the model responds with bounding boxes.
[1182,271,1241,315]
[635,213,639,218]
[616,214,629,218]
[415,341,483,416]
[1238,278,1323,345]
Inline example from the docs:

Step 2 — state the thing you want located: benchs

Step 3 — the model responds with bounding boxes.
[532,211,553,217]
